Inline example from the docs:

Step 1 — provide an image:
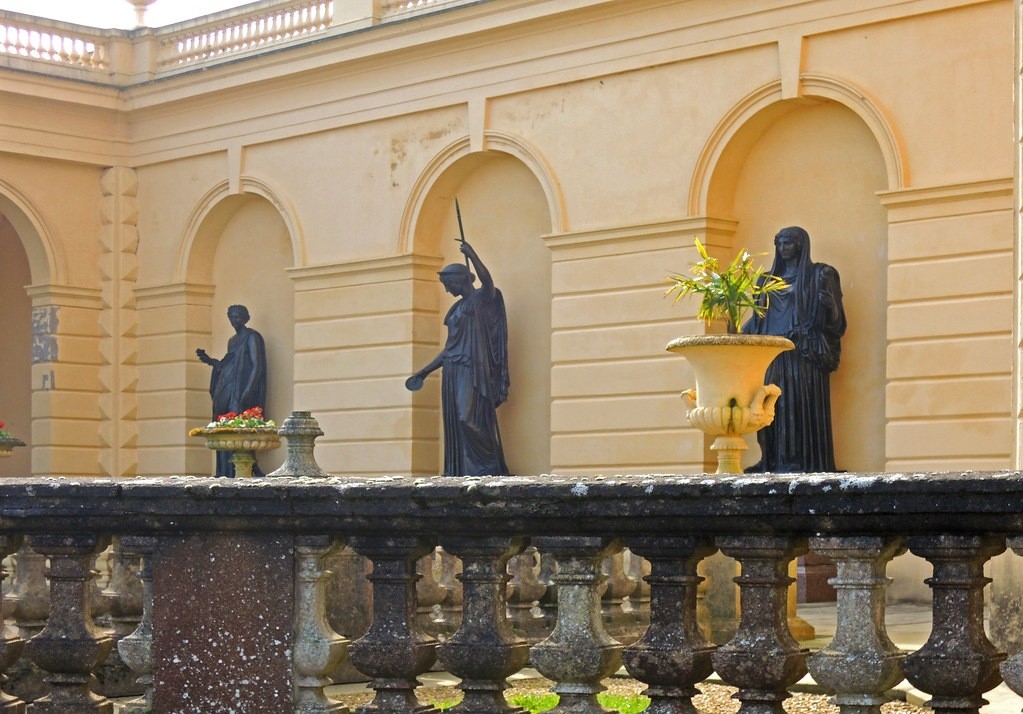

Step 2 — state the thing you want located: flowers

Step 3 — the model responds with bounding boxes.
[206,407,275,428]
[0,420,14,438]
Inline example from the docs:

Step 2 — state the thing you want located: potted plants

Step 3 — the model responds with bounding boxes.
[665,234,796,475]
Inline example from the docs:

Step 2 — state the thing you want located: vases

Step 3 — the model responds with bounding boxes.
[189,427,283,478]
[0,438,27,457]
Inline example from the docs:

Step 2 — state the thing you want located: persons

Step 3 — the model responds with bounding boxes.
[412,238,510,478]
[742,227,846,473]
[196,305,266,479]
